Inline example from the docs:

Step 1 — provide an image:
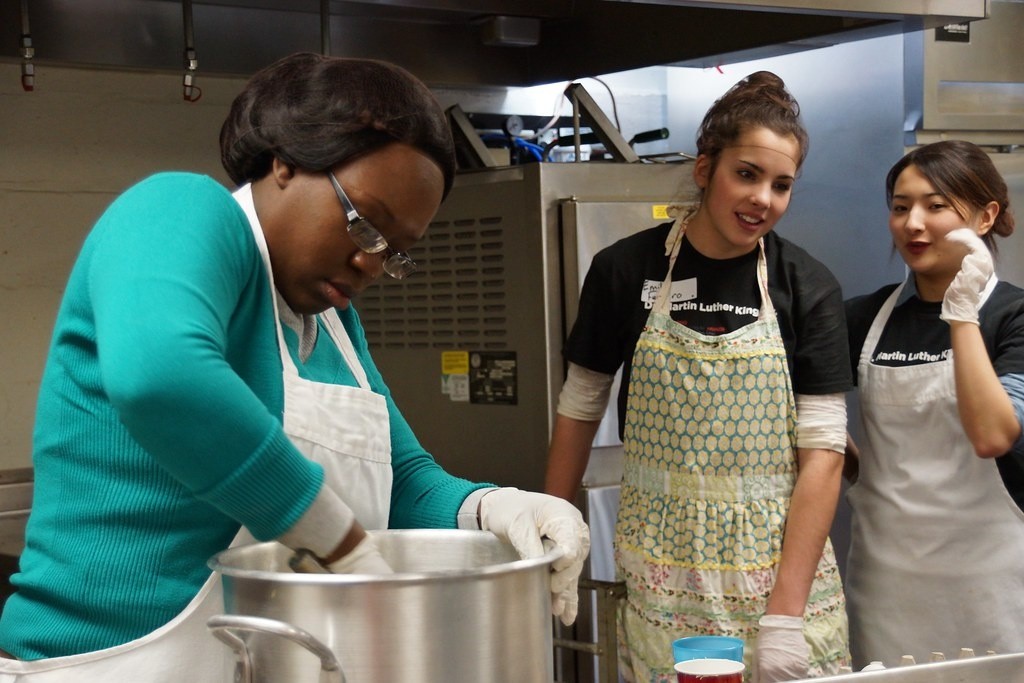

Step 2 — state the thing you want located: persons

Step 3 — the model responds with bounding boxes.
[841,139,1024,671]
[542,71,855,683]
[0,53,591,683]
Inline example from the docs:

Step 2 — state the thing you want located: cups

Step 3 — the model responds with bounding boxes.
[674,660,745,682]
[672,636,744,664]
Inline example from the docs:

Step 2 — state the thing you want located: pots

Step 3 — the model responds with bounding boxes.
[208,527,565,682]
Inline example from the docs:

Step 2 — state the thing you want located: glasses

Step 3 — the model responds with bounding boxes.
[327,171,419,282]
